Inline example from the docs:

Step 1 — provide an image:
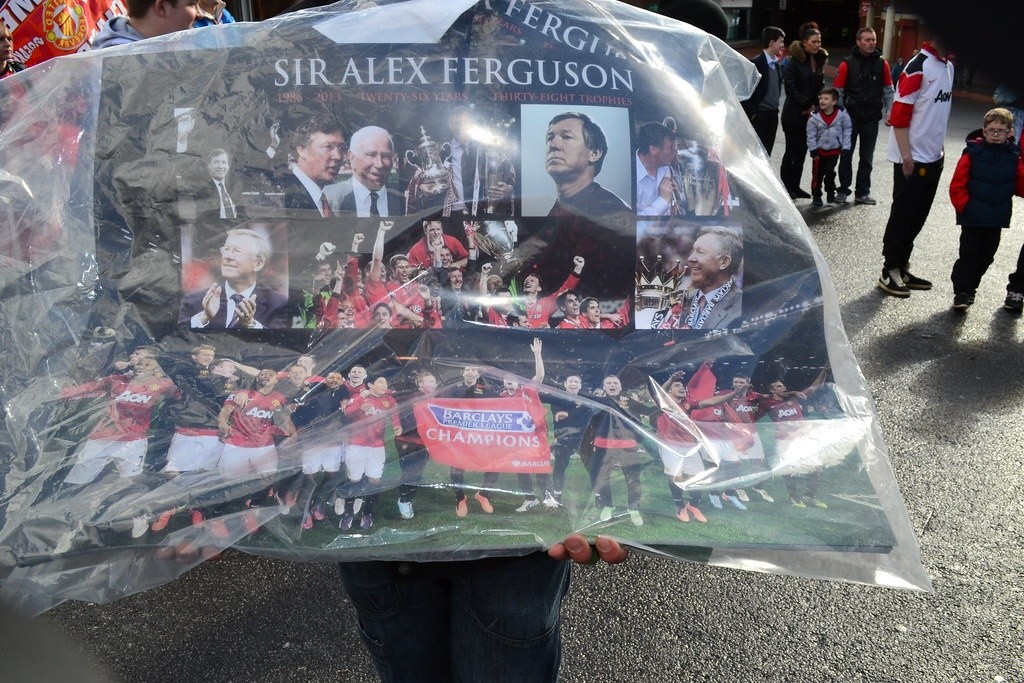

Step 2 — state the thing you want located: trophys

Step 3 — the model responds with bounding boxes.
[474,220,525,282]
[660,116,733,216]
[635,254,690,329]
[405,124,453,200]
[480,118,516,214]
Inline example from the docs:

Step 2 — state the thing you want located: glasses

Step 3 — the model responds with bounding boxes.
[985,128,1010,136]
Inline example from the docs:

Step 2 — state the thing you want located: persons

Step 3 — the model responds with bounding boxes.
[0,1,241,321]
[49,114,828,554]
[155,535,629,683]
[740,22,1024,310]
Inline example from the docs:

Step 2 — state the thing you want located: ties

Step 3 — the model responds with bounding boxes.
[370,192,380,219]
[226,293,245,328]
[320,194,335,217]
[218,183,235,218]
[698,296,706,314]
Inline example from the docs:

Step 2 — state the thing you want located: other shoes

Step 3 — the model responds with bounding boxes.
[812,197,823,205]
[791,188,811,199]
[828,193,834,203]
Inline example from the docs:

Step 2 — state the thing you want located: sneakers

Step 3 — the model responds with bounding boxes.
[1004,291,1024,311]
[953,295,976,309]
[835,193,847,203]
[877,266,911,296]
[51,489,828,554]
[900,268,933,288]
[855,195,876,204]
